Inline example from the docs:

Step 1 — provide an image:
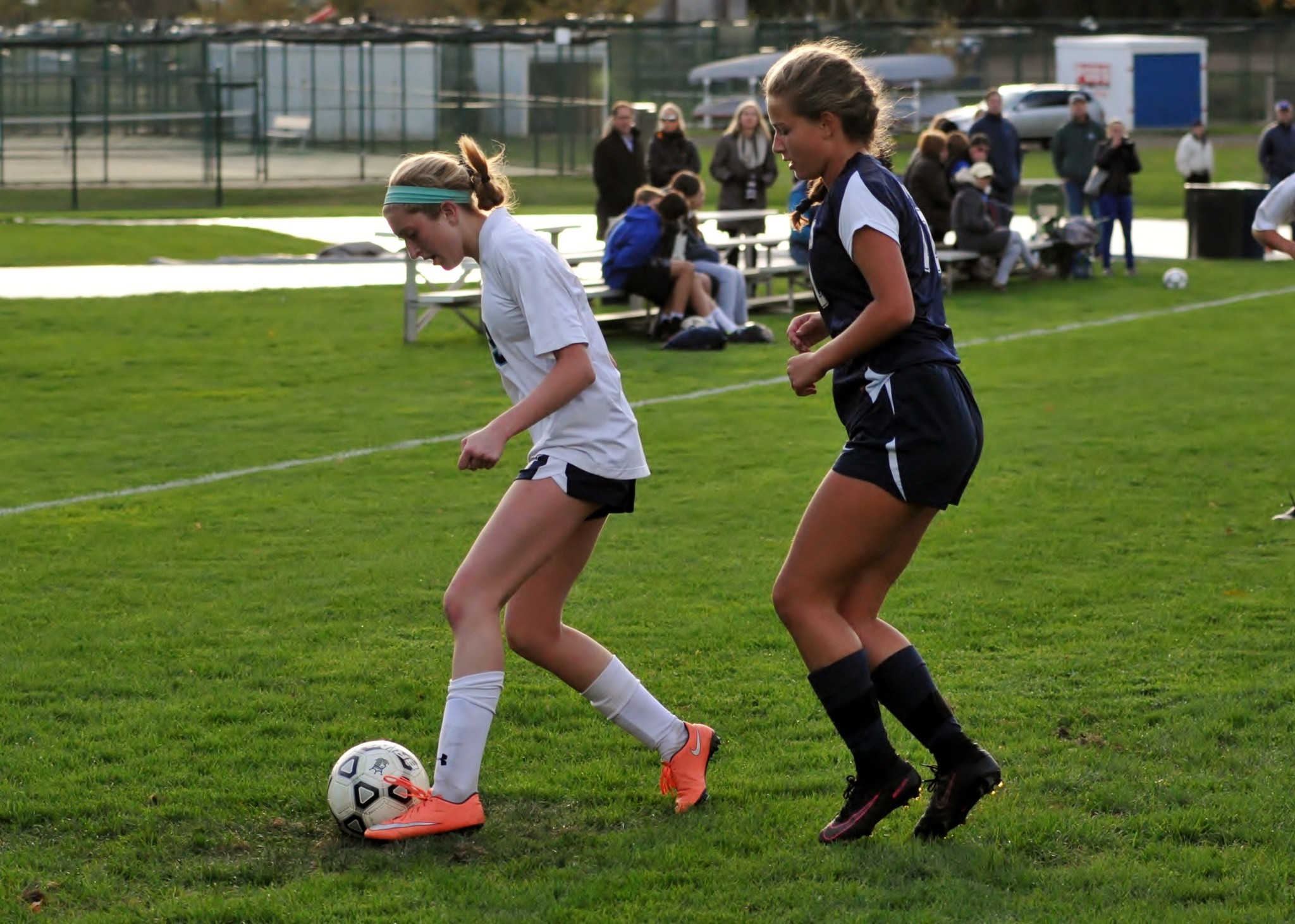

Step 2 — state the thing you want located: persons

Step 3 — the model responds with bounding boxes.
[647,102,702,191]
[789,91,1057,290]
[1049,92,1114,261]
[358,136,720,843]
[1250,167,1295,259]
[761,44,1004,845]
[1174,121,1215,188]
[602,170,776,353]
[707,102,779,297]
[591,99,646,241]
[1257,100,1295,254]
[1094,121,1142,278]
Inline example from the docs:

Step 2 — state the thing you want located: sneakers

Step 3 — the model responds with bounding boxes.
[659,721,721,815]
[362,775,485,843]
[913,744,1002,846]
[818,754,921,845]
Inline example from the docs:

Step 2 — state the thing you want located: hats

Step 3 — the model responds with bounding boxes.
[969,161,994,178]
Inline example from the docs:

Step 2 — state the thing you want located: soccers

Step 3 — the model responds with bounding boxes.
[324,740,433,837]
[1162,266,1189,291]
[680,314,709,329]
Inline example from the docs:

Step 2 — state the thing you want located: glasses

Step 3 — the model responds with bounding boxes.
[660,117,675,123]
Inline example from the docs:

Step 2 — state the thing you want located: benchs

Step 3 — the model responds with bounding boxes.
[394,208,1094,343]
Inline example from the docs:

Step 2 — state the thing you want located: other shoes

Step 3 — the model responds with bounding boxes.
[990,263,1139,295]
[652,315,759,344]
